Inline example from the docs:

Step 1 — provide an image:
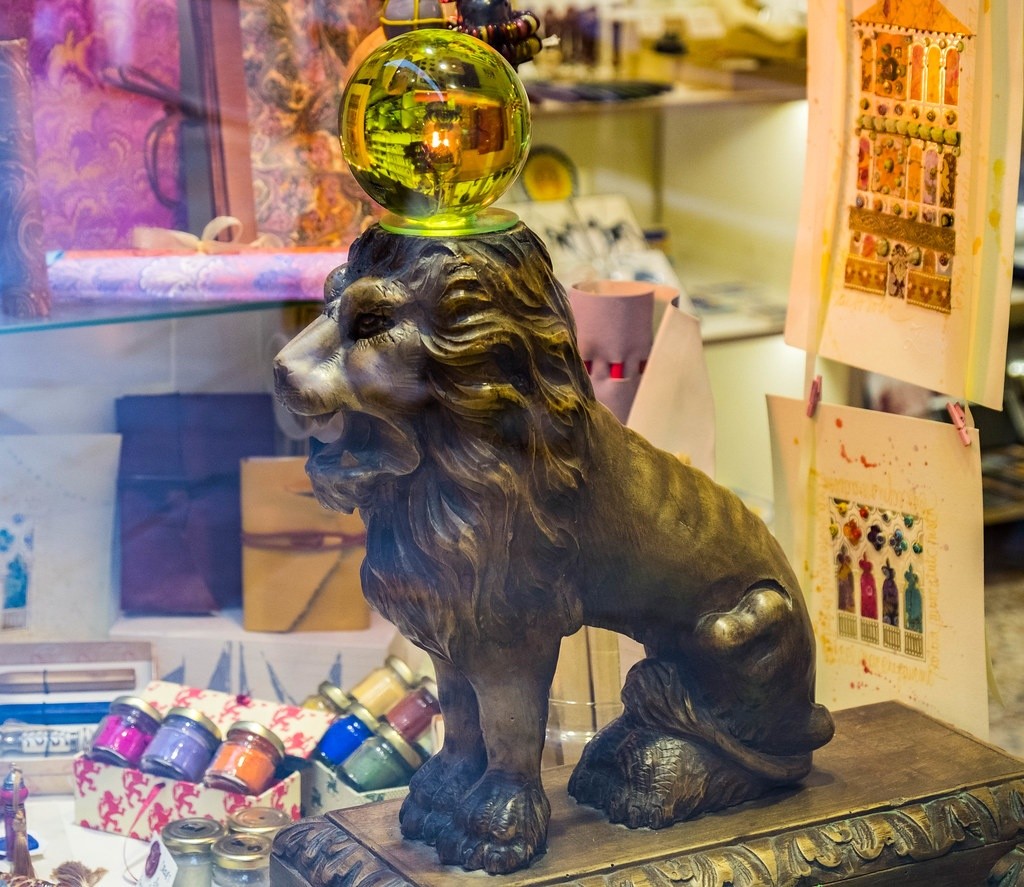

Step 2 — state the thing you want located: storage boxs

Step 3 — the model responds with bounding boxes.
[0,639,155,796]
[71,679,338,843]
[301,733,443,817]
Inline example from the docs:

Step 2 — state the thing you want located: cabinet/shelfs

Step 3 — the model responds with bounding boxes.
[523,82,811,350]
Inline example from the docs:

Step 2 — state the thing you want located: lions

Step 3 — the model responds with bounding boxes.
[269,212,837,877]
